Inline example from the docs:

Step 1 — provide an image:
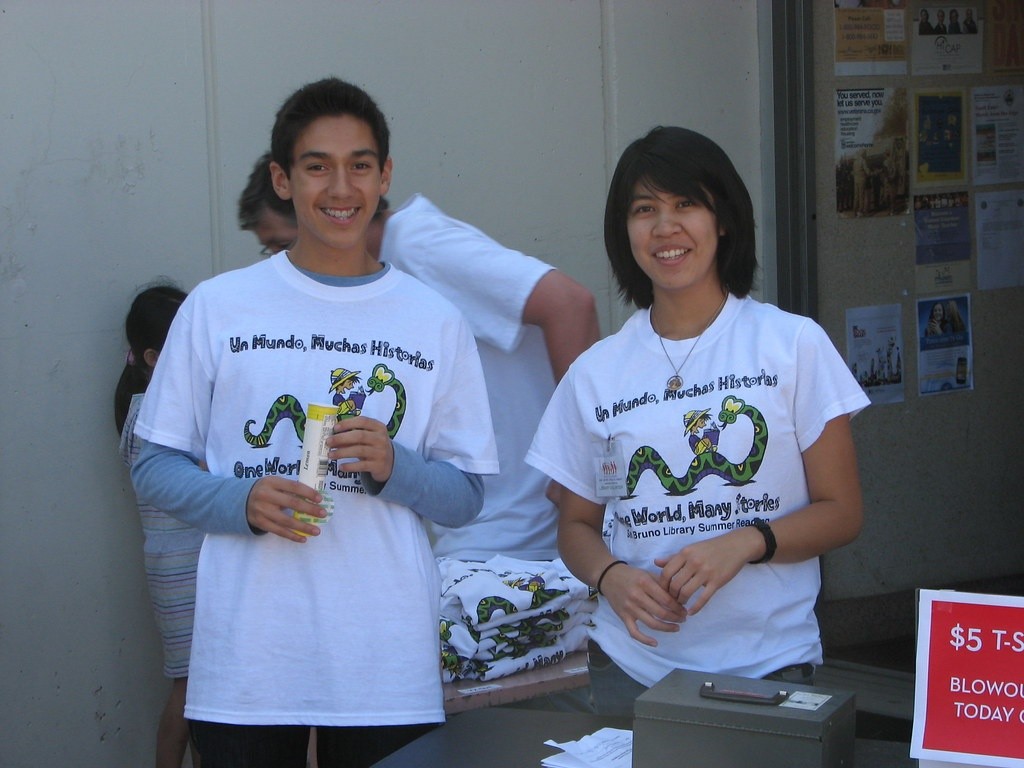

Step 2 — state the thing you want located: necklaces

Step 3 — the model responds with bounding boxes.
[653,289,727,390]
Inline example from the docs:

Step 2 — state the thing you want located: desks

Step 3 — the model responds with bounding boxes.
[371,707,918,768]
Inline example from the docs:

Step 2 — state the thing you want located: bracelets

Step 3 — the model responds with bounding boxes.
[747,519,777,565]
[597,560,627,596]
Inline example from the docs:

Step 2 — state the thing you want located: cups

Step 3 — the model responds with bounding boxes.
[294,401,341,537]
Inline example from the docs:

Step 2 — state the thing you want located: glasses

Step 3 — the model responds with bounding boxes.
[260,233,298,257]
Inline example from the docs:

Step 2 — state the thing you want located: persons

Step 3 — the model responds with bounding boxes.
[130,77,500,768]
[113,287,208,768]
[935,9,947,34]
[962,9,977,34]
[919,10,934,36]
[948,9,961,34]
[945,300,964,333]
[853,149,882,215]
[524,126,871,717]
[237,153,599,563]
[927,302,951,337]
